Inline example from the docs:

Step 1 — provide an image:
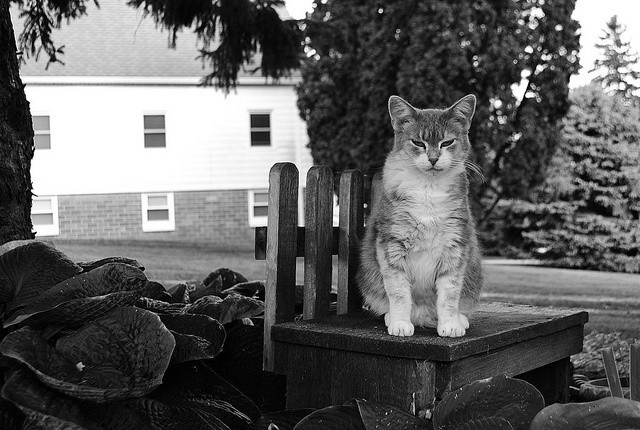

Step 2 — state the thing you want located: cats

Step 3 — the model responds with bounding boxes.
[358,94,486,337]
